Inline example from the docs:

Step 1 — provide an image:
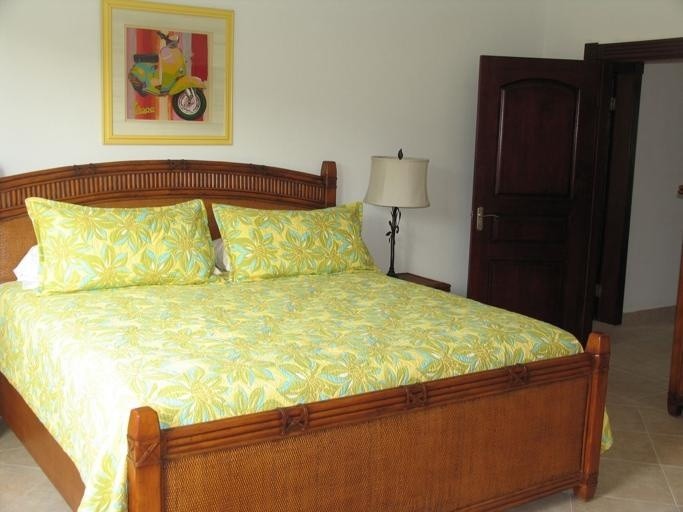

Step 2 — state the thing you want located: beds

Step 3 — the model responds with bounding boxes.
[0,158,615,512]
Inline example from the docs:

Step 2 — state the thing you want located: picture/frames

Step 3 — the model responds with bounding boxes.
[102,0,236,146]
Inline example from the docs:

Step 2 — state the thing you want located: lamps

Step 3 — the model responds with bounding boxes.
[362,149,430,275]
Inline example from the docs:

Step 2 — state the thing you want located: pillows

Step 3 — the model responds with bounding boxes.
[212,201,379,283]
[13,244,40,290]
[211,237,230,271]
[24,196,218,296]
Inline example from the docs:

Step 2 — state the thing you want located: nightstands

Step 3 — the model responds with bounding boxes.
[394,271,452,293]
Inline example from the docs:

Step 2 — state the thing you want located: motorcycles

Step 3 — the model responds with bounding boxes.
[129,31,209,121]
